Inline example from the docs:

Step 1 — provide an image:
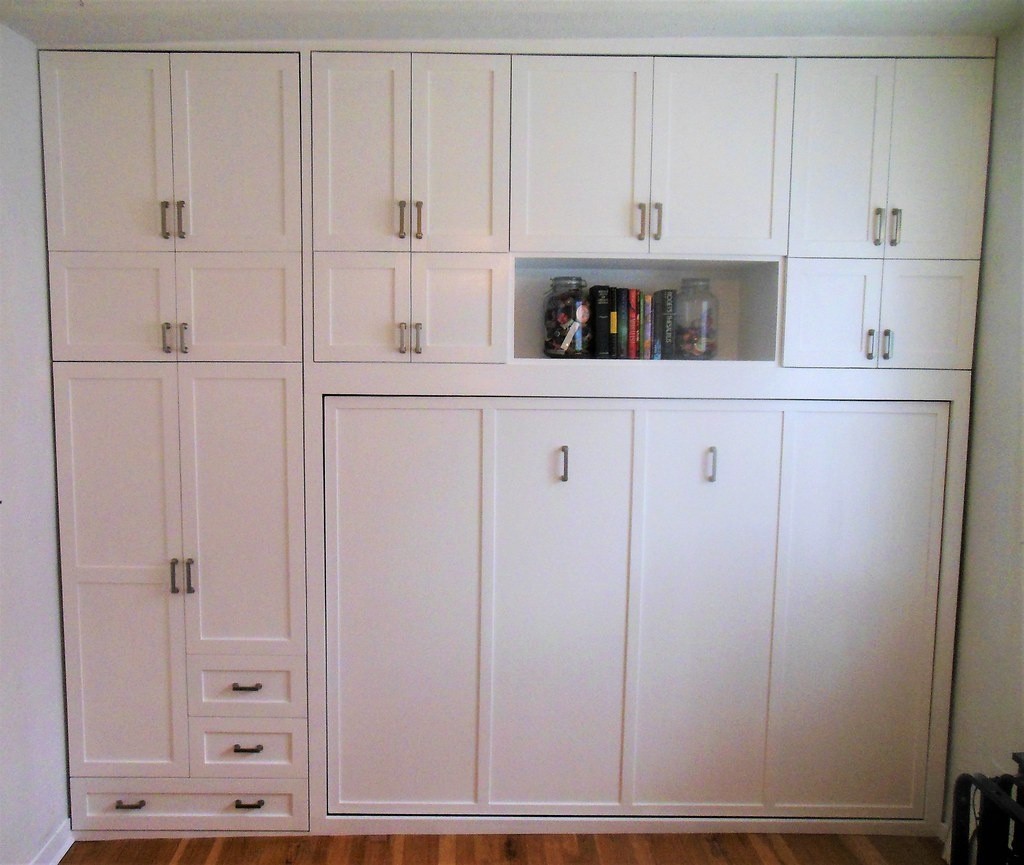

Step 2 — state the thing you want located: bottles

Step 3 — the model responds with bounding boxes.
[541,276,591,357]
[674,278,718,359]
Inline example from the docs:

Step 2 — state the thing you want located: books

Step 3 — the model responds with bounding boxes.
[588,285,677,360]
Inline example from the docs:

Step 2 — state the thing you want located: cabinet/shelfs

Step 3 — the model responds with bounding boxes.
[36,46,1000,839]
